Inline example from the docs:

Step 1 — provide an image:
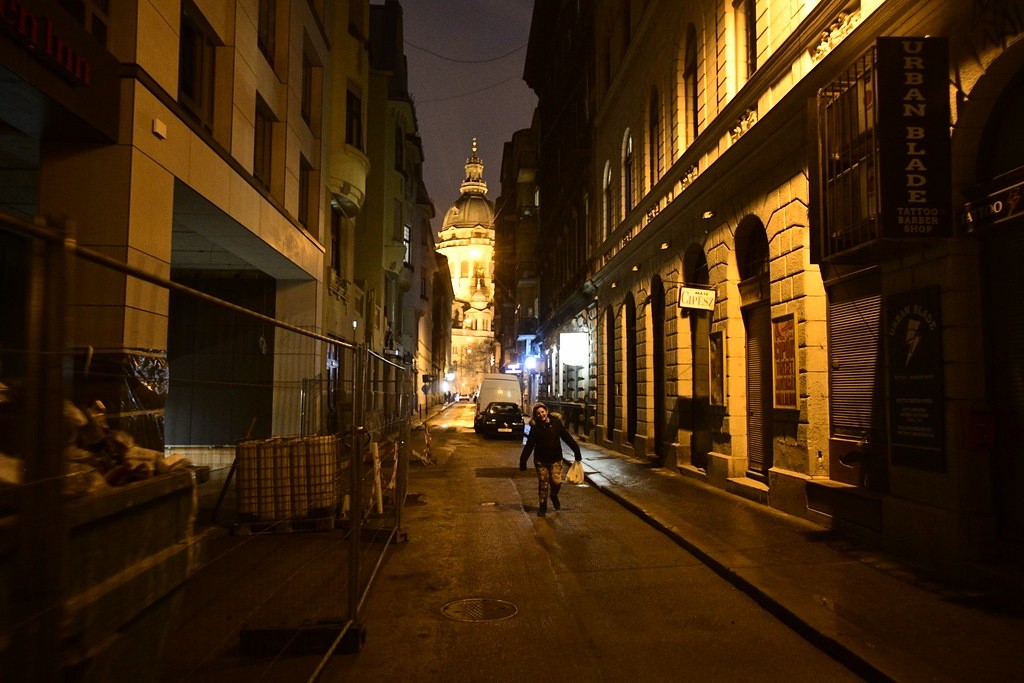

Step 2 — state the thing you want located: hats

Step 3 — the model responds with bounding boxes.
[533,402,548,412]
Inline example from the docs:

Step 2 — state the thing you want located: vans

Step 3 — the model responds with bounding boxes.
[474,373,525,441]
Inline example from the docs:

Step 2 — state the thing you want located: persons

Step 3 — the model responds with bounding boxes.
[520,402,582,516]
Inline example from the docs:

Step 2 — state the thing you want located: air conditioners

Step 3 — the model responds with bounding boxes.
[825,147,877,245]
[824,60,879,164]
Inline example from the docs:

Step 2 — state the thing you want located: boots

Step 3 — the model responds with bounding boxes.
[549,478,562,510]
[537,479,549,516]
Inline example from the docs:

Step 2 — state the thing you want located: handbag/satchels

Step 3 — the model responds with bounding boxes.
[565,460,584,484]
